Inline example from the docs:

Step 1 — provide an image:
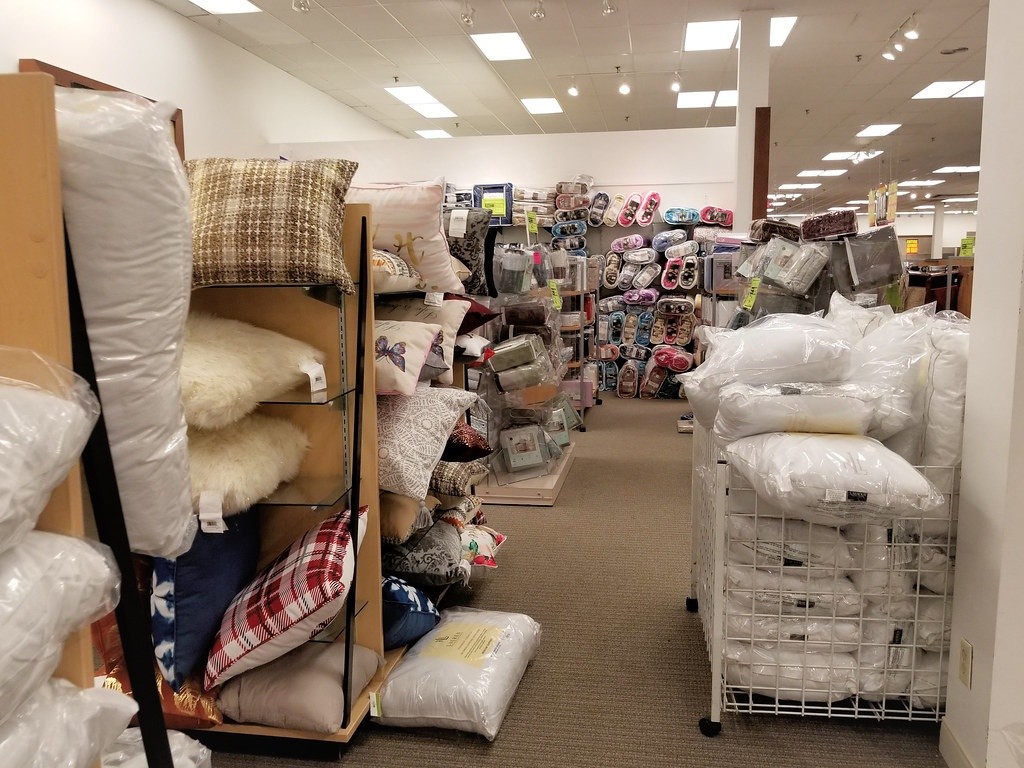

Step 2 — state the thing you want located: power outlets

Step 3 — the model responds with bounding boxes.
[958,636,973,690]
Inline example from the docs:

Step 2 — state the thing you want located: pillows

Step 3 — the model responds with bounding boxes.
[0,526,122,727]
[443,207,493,297]
[186,414,311,517]
[375,294,506,590]
[382,574,442,652]
[182,159,359,295]
[216,642,388,735]
[451,255,473,281]
[204,505,370,692]
[91,613,223,730]
[147,507,263,694]
[675,291,971,706]
[345,176,465,295]
[53,84,199,558]
[373,248,420,294]
[179,311,326,431]
[369,604,542,742]
[0,345,102,556]
[0,675,139,768]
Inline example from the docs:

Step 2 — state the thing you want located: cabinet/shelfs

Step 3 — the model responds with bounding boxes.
[188,203,405,763]
[527,261,603,433]
